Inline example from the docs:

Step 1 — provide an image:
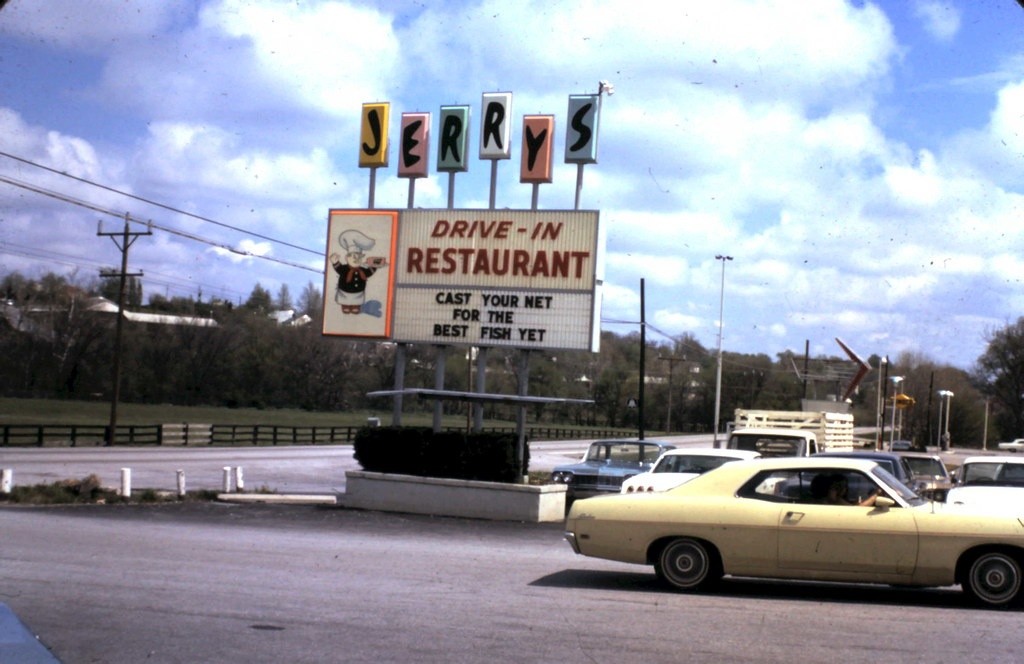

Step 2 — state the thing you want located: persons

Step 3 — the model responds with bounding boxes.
[809,474,881,506]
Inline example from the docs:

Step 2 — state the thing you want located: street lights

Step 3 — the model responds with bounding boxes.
[889,375,904,449]
[936,390,953,450]
[714,254,732,447]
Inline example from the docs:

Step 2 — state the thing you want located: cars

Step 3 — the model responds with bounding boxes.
[784,451,917,508]
[893,452,953,504]
[997,438,1024,452]
[890,441,927,452]
[565,457,1024,609]
[553,438,680,513]
[620,447,760,497]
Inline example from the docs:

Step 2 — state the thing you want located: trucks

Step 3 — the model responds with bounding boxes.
[726,407,854,459]
